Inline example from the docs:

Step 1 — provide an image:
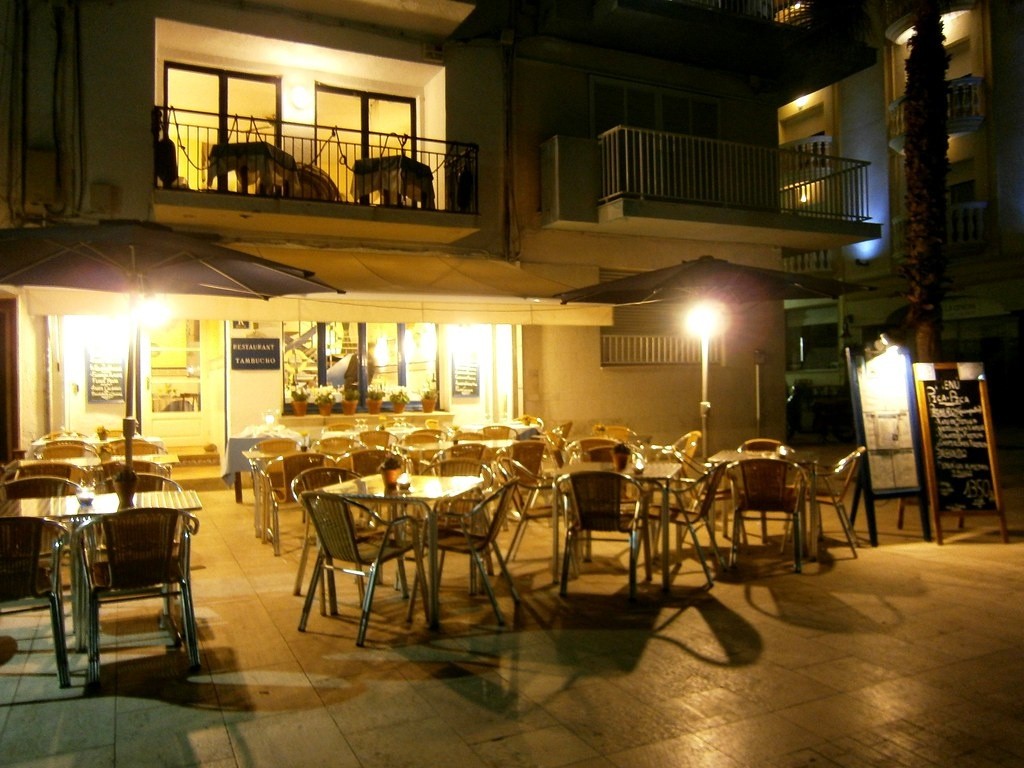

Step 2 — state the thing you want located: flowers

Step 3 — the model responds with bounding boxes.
[314,384,338,405]
[286,386,310,401]
[390,385,410,404]
[366,374,386,399]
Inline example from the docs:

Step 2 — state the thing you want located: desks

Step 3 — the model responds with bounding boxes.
[0,491,202,669]
[708,449,825,563]
[242,446,394,544]
[313,474,484,630]
[321,428,448,444]
[447,420,540,438]
[398,439,518,531]
[221,436,305,503]
[548,461,683,591]
[5,452,181,491]
[31,435,162,459]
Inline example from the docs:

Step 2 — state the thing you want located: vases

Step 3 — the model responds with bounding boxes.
[291,401,307,416]
[365,398,382,414]
[318,403,332,415]
[393,403,406,414]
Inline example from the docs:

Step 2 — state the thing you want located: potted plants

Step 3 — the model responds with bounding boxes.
[113,469,139,501]
[611,444,631,470]
[420,386,437,413]
[340,389,360,414]
[96,426,109,440]
[377,455,402,488]
[98,443,116,460]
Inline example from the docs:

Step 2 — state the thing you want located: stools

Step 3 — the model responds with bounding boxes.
[207,142,301,198]
[350,155,436,211]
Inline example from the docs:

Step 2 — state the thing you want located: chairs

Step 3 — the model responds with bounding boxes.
[0,462,87,495]
[243,414,867,647]
[31,446,100,457]
[256,163,342,203]
[99,443,169,456]
[75,473,185,654]
[0,476,83,652]
[72,506,199,682]
[86,460,172,495]
[0,516,70,690]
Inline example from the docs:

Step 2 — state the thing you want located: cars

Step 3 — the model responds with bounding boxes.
[796,382,851,405]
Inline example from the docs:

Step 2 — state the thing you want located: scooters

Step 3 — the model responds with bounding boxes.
[786,387,855,445]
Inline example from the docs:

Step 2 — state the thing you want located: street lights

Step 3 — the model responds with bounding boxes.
[832,226,885,363]
